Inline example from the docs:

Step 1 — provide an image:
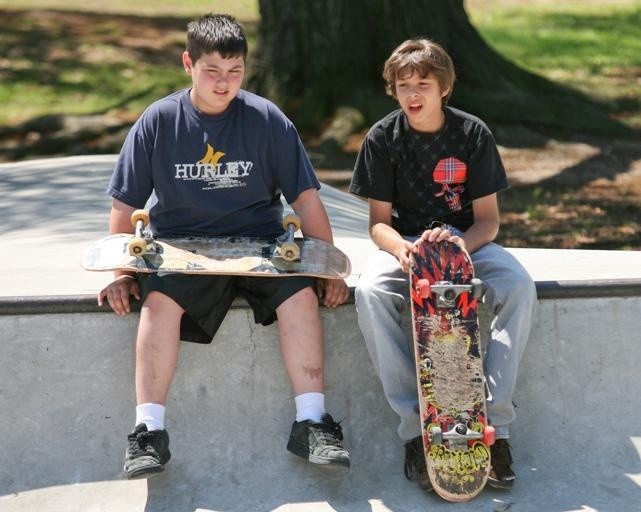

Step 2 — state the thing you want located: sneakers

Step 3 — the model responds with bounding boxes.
[287,414,351,465]
[123,423,171,477]
[405,435,434,492]
[487,439,516,489]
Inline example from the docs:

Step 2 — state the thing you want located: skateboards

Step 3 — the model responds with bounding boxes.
[82,209,351,280]
[411,235,497,502]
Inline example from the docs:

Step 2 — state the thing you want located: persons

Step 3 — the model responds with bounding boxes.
[347,37,539,493]
[97,13,352,480]
